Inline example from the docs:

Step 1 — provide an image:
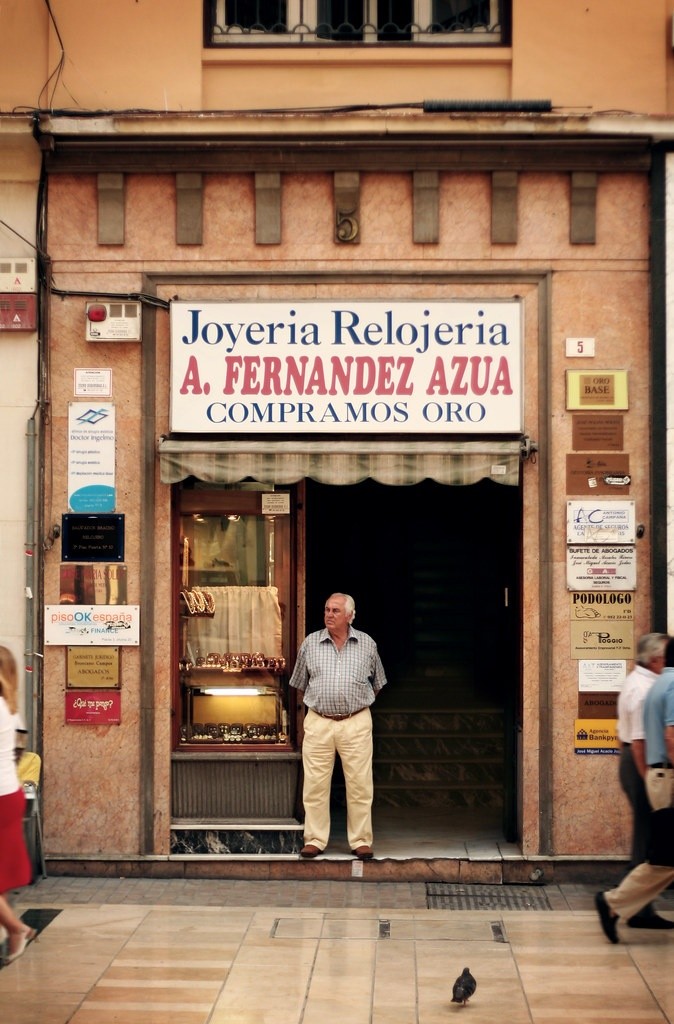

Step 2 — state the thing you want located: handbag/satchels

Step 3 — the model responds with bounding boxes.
[643,807,674,867]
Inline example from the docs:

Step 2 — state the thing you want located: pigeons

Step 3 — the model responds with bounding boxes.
[450,967,477,1009]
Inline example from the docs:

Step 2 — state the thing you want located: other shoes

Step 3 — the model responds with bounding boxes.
[594,891,627,944]
[352,846,375,858]
[301,845,325,858]
[625,903,674,929]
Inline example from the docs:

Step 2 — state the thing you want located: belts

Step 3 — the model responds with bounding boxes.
[313,707,368,721]
[650,763,673,769]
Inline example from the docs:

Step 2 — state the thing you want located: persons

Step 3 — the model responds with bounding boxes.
[0,647,41,966]
[594,632,674,944]
[289,593,388,859]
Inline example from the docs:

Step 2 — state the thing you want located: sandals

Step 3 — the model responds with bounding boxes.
[0,928,38,965]
